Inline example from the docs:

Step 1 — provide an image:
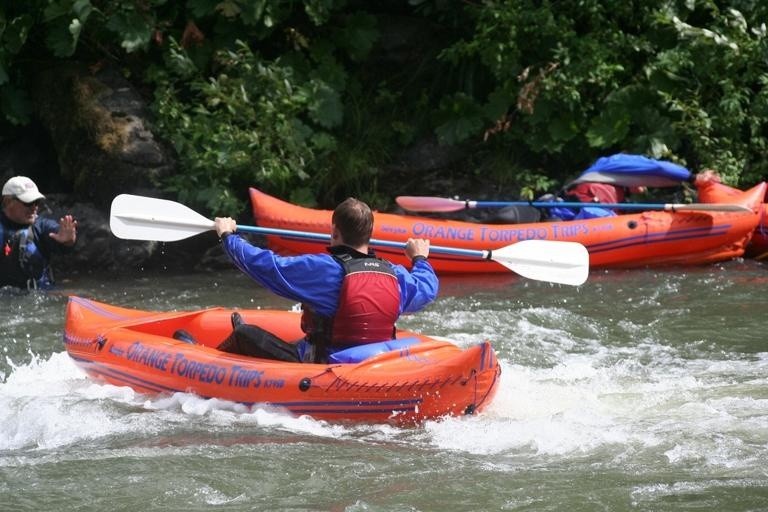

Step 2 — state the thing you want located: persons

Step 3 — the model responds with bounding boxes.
[0,175,77,290]
[171,197,439,363]
[391,152,719,228]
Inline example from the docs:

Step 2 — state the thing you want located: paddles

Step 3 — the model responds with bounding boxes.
[396,195,749,213]
[110,194,589,287]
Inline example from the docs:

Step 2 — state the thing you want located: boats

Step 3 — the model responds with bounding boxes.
[62,293,502,426]
[246,183,765,271]
[695,168,768,250]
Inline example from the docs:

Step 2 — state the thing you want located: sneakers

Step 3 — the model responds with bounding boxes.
[231,312,243,330]
[173,329,195,342]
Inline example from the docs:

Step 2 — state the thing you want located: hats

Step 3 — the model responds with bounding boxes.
[2,175,46,204]
